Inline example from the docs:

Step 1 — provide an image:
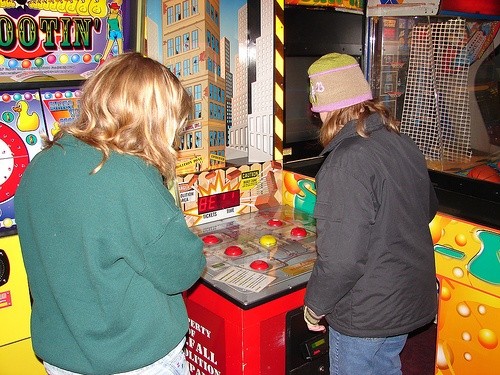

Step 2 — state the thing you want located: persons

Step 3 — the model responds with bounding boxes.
[302,51,443,375]
[13,52,206,375]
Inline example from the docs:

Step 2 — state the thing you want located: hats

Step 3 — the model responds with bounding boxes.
[308,52,372,112]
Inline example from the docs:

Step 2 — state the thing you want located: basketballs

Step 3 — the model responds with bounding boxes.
[467,166,500,182]
